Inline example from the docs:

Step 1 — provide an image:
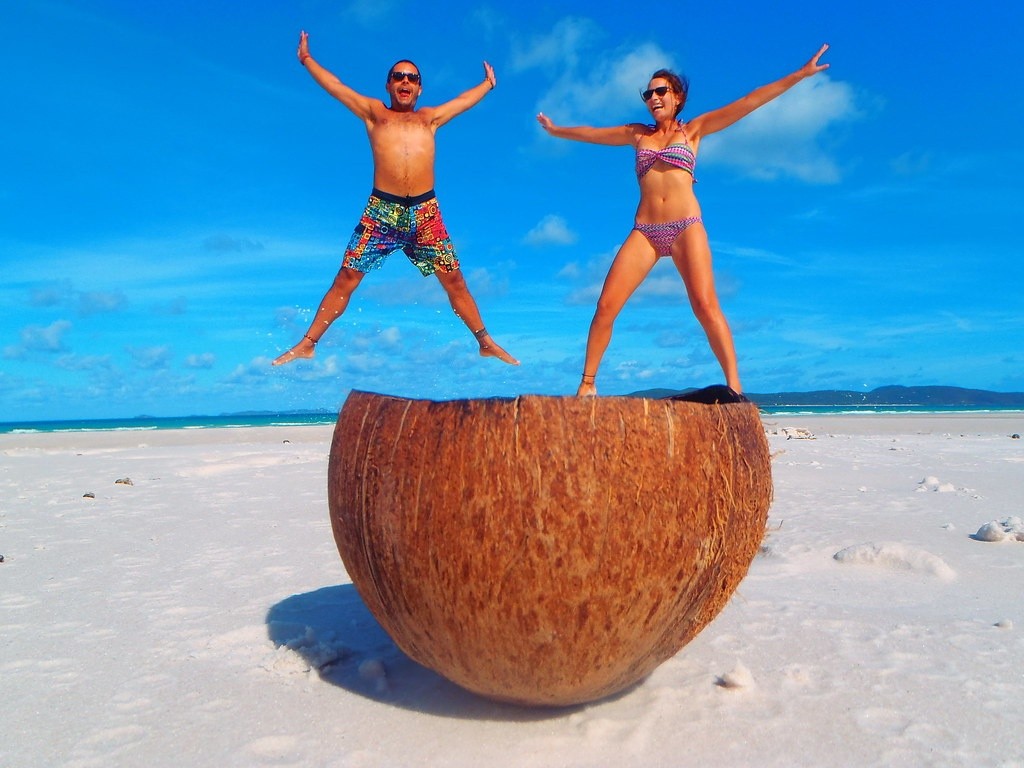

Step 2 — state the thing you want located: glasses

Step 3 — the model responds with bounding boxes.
[389,72,420,82]
[643,87,674,100]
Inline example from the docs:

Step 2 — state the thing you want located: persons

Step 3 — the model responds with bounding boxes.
[537,44,830,399]
[272,29,520,366]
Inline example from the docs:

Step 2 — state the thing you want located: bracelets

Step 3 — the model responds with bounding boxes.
[485,78,493,90]
[300,54,311,65]
